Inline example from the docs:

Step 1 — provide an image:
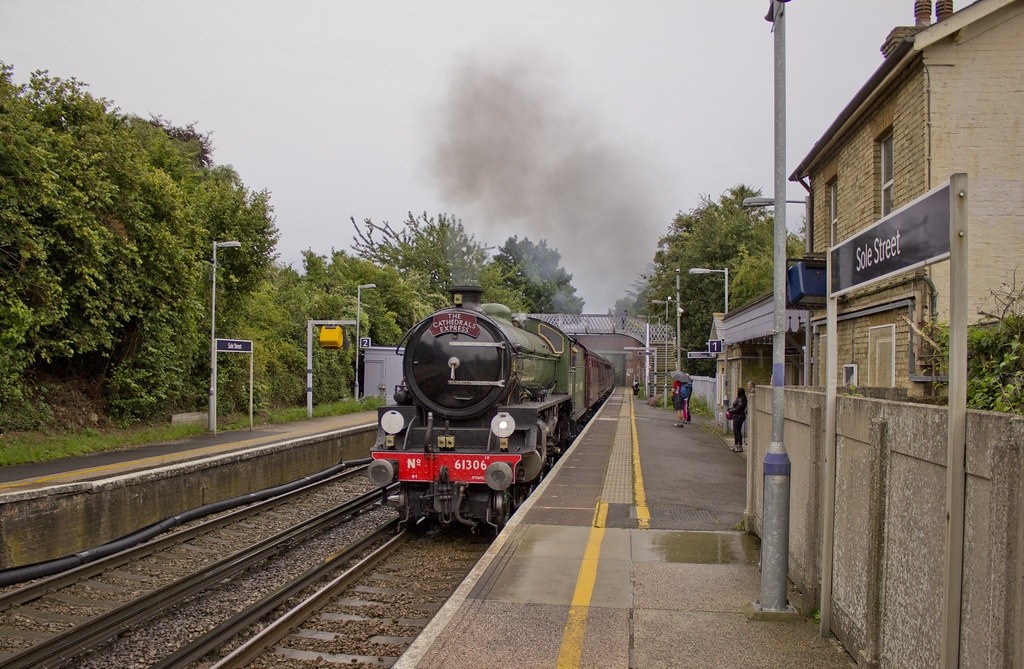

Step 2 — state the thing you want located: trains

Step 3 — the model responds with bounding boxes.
[369,285,616,539]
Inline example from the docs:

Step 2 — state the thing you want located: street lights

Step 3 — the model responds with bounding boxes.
[688,268,730,436]
[209,241,242,433]
[652,300,668,409]
[742,194,814,385]
[355,283,379,401]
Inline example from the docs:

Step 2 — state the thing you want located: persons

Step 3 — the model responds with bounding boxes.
[728,387,747,451]
[748,381,755,394]
[670,373,693,427]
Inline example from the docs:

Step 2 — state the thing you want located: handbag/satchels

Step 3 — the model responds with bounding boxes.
[680,382,689,399]
[726,409,733,421]
[672,392,683,410]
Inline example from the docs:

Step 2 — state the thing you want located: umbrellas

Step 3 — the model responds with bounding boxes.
[671,370,690,382]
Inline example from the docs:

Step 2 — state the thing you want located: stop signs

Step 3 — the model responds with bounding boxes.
[217,340,250,351]
[828,179,950,298]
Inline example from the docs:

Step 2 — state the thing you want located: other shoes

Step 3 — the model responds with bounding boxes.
[729,444,738,450]
[734,445,743,452]
[673,423,683,427]
